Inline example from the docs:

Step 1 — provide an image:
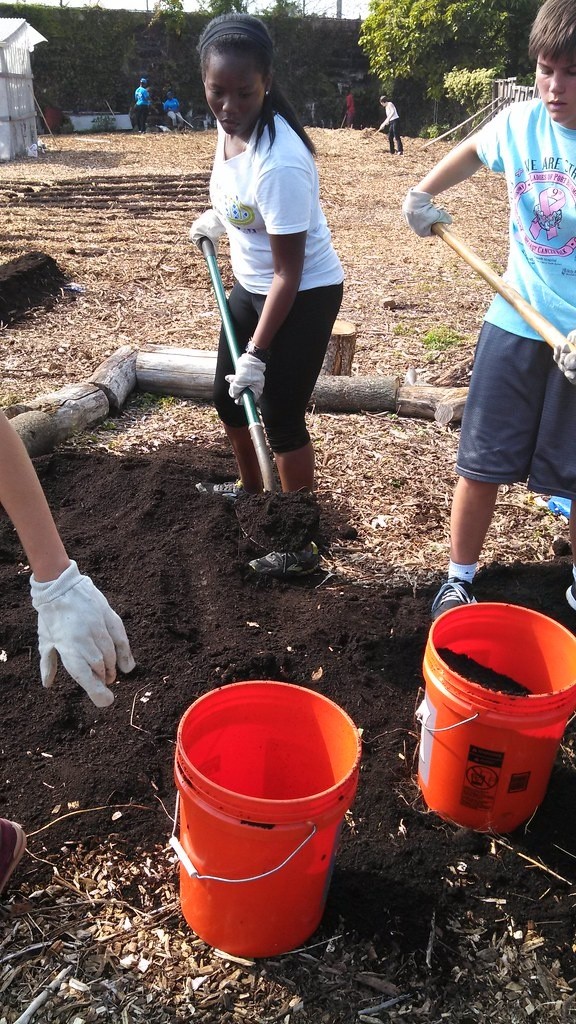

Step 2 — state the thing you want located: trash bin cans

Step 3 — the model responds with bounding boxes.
[45,106,61,133]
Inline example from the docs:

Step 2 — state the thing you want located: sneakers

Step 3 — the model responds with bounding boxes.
[566,581,576,611]
[431,576,478,625]
[248,541,319,575]
[195,479,250,498]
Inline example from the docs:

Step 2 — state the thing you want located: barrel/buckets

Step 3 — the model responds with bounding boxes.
[418,603,576,833]
[169,681,360,959]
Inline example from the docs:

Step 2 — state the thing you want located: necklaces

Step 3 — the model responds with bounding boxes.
[242,139,249,152]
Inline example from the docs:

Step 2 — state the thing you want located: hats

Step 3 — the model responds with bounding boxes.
[166,92,174,96]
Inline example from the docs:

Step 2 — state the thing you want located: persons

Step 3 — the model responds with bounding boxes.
[402,0,576,623]
[379,95,403,155]
[0,407,137,896]
[163,90,184,130]
[133,77,153,133]
[345,89,355,127]
[188,13,345,578]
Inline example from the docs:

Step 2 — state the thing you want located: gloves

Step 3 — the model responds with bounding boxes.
[402,187,452,237]
[224,352,265,406]
[552,331,576,385]
[29,559,136,708]
[189,209,226,259]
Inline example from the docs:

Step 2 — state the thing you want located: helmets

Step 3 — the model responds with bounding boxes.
[140,78,148,85]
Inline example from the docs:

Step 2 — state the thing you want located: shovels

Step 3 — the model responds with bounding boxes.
[200,236,319,555]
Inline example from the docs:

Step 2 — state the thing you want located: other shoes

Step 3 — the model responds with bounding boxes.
[0,818,27,894]
[397,151,404,155]
